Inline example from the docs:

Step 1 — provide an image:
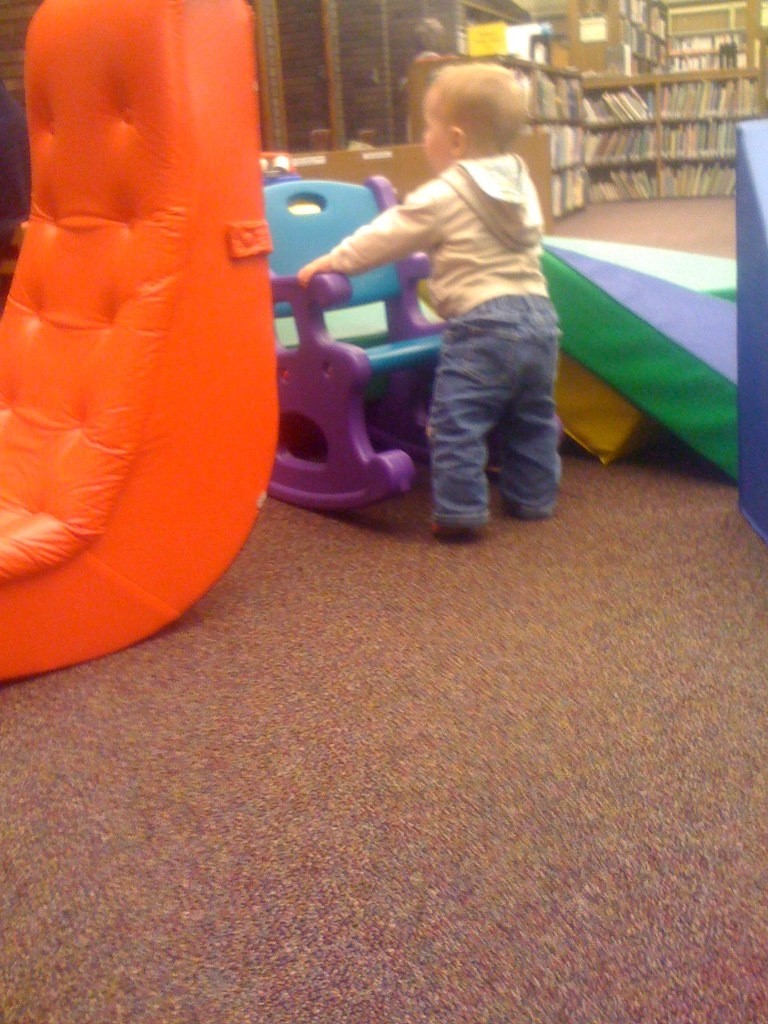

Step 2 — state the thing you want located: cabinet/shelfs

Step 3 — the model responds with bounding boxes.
[407,0,768,222]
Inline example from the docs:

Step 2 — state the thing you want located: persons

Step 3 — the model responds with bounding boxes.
[298,61,560,535]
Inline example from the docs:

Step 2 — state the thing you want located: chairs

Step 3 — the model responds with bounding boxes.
[0,0,278,684]
[262,172,504,511]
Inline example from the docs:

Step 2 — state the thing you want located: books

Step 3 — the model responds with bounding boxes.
[602,0,670,77]
[510,70,585,218]
[661,78,760,197]
[583,85,658,202]
[669,32,748,74]
